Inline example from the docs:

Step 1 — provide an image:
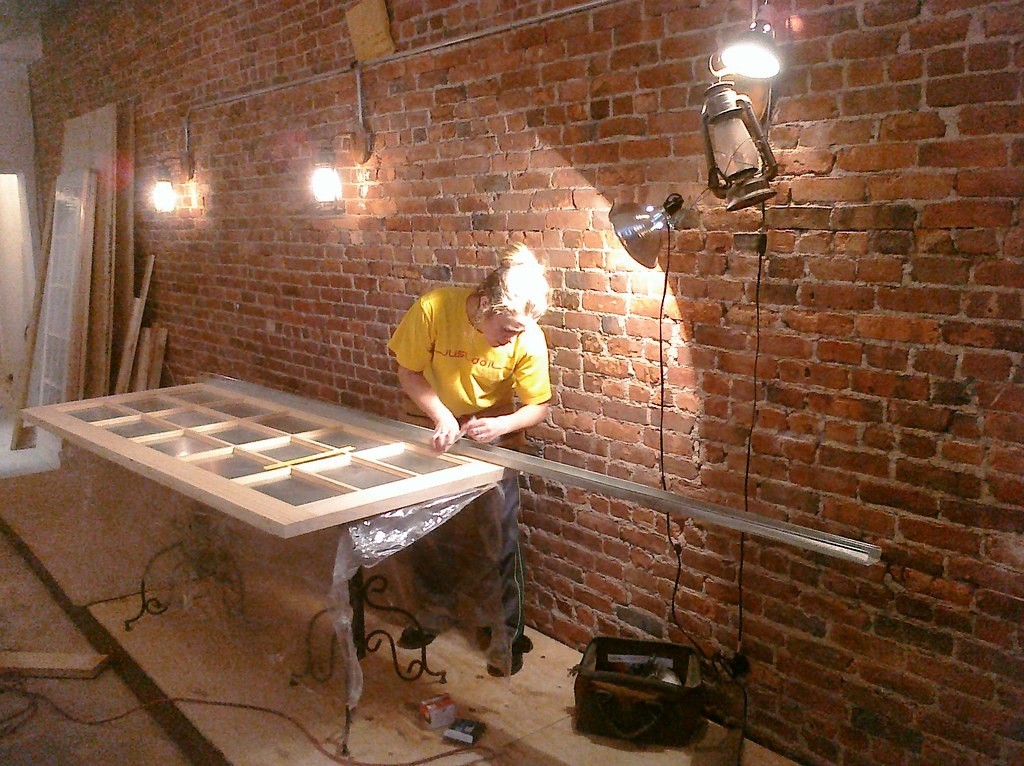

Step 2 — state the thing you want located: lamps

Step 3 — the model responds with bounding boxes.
[309,68,372,217]
[152,118,196,213]
[721,4,784,80]
[700,81,778,212]
[610,191,748,681]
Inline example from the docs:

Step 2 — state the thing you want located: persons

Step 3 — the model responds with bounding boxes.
[387,241,552,675]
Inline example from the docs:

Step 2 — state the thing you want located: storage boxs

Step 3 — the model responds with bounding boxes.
[419,696,456,729]
[444,718,486,747]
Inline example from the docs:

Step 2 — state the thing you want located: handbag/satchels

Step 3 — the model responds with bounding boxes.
[573,638,708,748]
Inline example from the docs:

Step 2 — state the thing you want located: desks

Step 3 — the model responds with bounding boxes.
[123,483,510,757]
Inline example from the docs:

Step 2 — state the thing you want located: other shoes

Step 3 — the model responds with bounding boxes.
[486,635,533,675]
[403,607,455,645]
